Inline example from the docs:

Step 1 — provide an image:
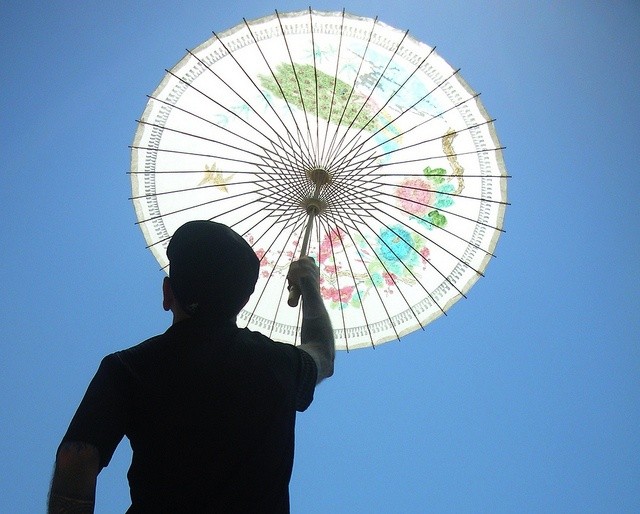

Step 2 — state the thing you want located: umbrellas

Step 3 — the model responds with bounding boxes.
[123,7,511,354]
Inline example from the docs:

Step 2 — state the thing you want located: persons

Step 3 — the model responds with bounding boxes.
[45,220,336,509]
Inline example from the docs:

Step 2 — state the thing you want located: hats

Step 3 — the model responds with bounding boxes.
[166,221,260,297]
